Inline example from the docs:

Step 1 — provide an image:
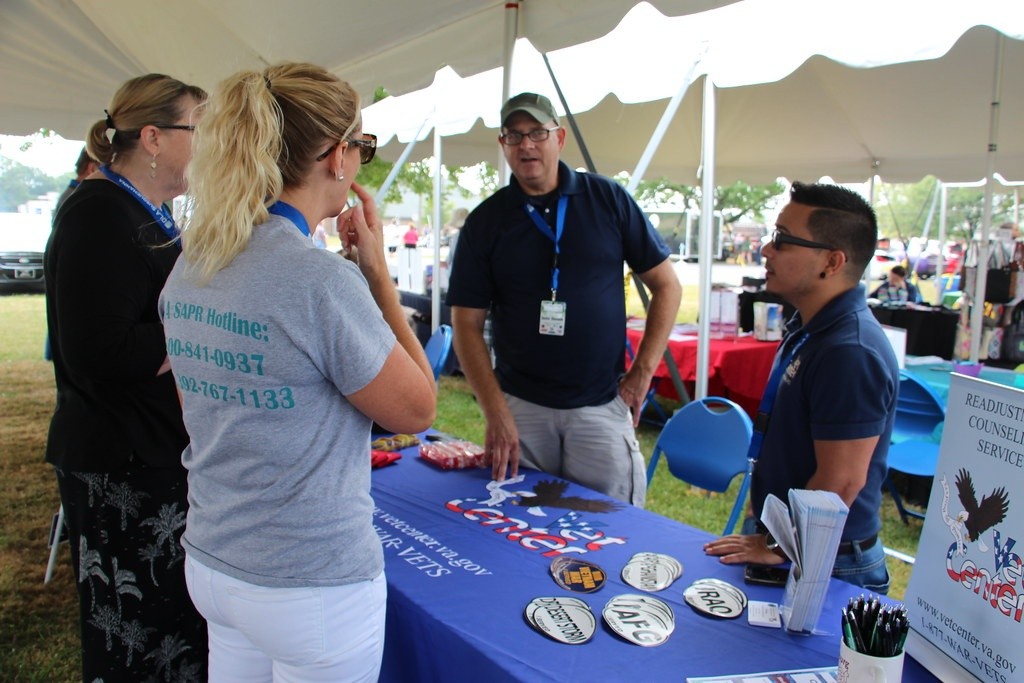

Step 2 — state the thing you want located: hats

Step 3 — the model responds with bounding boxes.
[501,93,558,128]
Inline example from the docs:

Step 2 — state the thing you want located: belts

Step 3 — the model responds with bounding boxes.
[838,533,878,555]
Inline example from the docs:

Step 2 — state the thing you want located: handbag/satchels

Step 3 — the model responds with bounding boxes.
[953,240,1024,364]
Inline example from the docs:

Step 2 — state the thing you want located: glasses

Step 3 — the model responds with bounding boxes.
[499,126,560,147]
[771,229,848,265]
[317,133,377,164]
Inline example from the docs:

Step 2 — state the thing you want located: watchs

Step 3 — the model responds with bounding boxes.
[764,531,792,563]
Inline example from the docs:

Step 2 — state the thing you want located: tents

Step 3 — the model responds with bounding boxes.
[2,3,736,143]
[363,0,1023,404]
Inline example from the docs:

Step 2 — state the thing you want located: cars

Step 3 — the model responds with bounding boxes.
[910,254,946,278]
[866,252,901,279]
[0,212,50,287]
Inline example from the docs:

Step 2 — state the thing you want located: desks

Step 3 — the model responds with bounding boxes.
[625,317,783,413]
[370,429,940,683]
[736,290,959,361]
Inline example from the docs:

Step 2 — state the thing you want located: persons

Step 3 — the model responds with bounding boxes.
[868,265,917,303]
[40,62,439,683]
[444,92,683,510]
[703,178,901,596]
[732,228,767,267]
[387,217,431,254]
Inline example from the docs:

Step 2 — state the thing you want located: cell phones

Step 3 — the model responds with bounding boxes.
[744,565,790,587]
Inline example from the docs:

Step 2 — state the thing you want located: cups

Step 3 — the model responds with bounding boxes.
[838,636,905,683]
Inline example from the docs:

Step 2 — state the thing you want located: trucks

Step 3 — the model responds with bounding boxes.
[643,210,722,261]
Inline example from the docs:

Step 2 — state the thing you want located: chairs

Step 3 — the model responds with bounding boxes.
[645,396,755,535]
[425,324,453,379]
[888,369,946,528]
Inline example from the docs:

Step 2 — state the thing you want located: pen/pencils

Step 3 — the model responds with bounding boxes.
[842,593,910,658]
